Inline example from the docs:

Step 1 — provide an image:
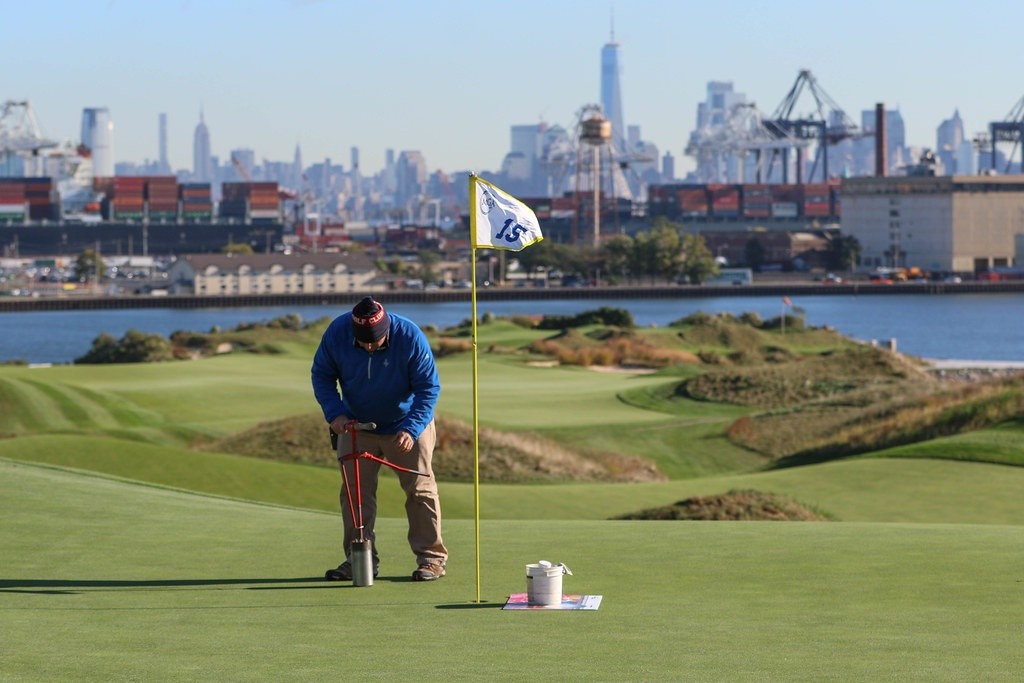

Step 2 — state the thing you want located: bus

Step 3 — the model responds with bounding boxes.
[700,268,753,286]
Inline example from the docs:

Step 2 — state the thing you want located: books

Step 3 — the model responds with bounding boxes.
[502,592,604,611]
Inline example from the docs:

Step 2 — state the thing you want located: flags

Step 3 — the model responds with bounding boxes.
[466,175,545,252]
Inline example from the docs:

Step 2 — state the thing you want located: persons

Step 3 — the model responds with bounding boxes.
[310,295,450,581]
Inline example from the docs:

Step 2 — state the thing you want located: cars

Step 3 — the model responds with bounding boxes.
[870,265,1005,282]
[822,273,842,284]
[382,270,596,289]
[1,260,170,283]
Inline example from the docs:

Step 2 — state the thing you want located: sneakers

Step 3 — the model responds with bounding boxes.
[412,563,445,581]
[325,561,378,580]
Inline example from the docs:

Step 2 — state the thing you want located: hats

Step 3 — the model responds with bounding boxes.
[352,296,389,343]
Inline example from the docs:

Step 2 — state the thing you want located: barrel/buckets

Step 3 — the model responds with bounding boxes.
[526,563,563,606]
